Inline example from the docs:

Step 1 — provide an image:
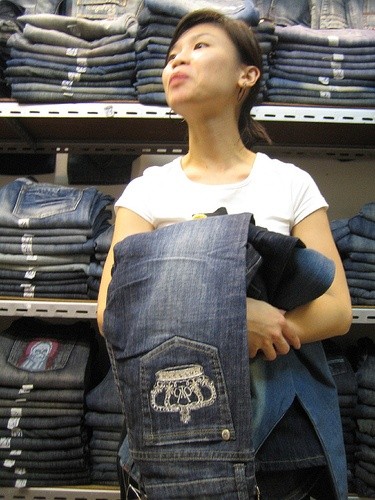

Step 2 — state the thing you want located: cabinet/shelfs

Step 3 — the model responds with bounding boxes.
[0,95,375,500]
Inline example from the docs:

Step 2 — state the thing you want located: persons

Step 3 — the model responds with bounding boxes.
[95,9,353,499]
